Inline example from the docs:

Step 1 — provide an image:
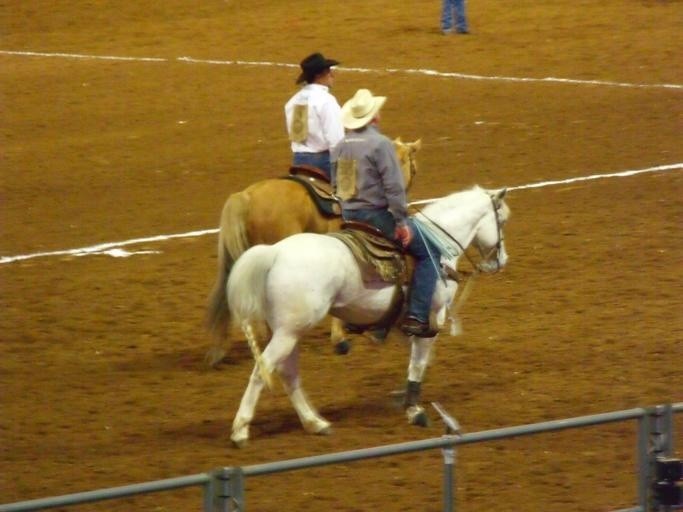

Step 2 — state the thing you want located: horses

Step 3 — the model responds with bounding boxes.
[200,135,423,368]
[225,183,511,449]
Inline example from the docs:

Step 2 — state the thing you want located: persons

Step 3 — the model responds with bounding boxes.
[330,88,440,337]
[283,53,345,177]
[440,0,469,34]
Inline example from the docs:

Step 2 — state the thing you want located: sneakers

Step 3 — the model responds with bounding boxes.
[401,317,438,338]
[443,26,469,35]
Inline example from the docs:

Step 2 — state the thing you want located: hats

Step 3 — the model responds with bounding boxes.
[296,53,339,85]
[339,88,386,130]
